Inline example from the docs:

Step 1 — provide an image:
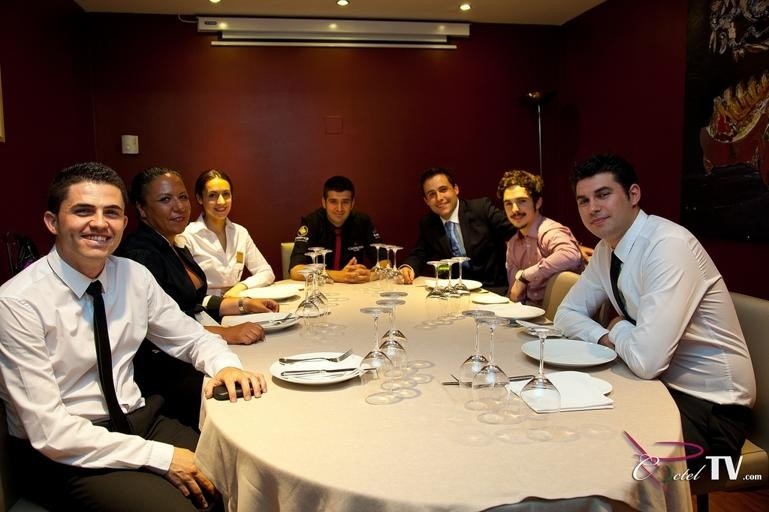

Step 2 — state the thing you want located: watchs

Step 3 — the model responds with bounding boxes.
[515,268,530,285]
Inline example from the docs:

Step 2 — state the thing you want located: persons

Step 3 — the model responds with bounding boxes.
[494,164,587,309]
[549,150,759,477]
[0,159,266,512]
[287,174,394,283]
[116,165,281,422]
[173,167,276,296]
[395,166,593,293]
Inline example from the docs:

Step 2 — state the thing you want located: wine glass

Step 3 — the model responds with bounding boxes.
[295,245,331,340]
[361,289,408,405]
[458,310,563,444]
[424,257,472,327]
[366,244,406,304]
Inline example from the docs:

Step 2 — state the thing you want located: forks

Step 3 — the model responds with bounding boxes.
[278,347,353,363]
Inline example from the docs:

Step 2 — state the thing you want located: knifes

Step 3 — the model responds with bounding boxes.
[282,367,376,374]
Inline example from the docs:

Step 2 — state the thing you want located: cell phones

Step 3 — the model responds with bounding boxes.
[213,384,263,401]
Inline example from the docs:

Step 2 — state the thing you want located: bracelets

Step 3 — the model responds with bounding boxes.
[239,282,248,290]
[236,297,254,315]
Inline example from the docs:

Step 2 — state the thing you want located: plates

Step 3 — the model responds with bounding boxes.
[427,278,481,291]
[483,304,545,319]
[224,310,299,332]
[523,338,619,366]
[241,286,298,300]
[270,352,368,384]
[471,294,509,304]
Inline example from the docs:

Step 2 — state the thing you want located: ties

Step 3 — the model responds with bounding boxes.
[87,280,127,434]
[333,229,341,270]
[446,221,469,268]
[610,251,636,324]
